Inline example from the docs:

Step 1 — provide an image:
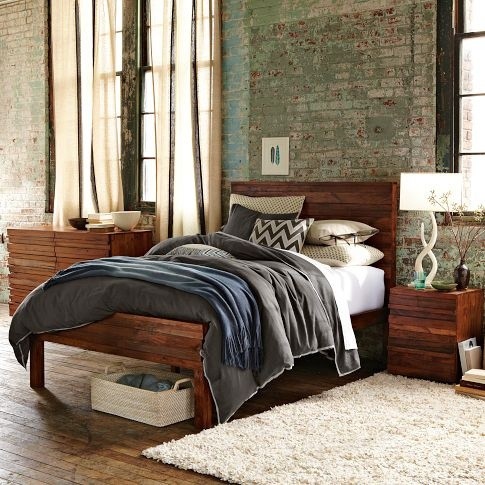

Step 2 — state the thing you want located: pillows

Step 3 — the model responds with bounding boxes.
[300,245,385,268]
[303,219,378,246]
[222,204,316,261]
[229,194,306,223]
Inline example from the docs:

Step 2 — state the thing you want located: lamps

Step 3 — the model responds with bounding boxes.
[400,170,466,289]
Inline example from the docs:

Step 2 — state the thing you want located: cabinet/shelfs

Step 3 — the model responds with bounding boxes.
[386,286,484,384]
[6,225,154,317]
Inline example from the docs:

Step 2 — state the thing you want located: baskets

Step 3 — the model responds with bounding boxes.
[91,362,195,428]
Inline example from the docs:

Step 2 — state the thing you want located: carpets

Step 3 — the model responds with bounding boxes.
[139,371,484,485]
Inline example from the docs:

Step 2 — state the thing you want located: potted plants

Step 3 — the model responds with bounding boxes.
[426,161,485,289]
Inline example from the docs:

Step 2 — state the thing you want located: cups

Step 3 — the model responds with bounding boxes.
[415,271,428,289]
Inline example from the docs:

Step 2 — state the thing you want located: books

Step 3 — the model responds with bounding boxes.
[457,336,482,378]
[85,212,115,234]
[454,367,485,400]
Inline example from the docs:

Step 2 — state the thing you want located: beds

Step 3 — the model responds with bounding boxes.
[9,179,397,438]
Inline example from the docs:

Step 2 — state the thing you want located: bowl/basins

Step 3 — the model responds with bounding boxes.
[431,280,459,292]
[68,218,88,230]
[111,210,142,231]
